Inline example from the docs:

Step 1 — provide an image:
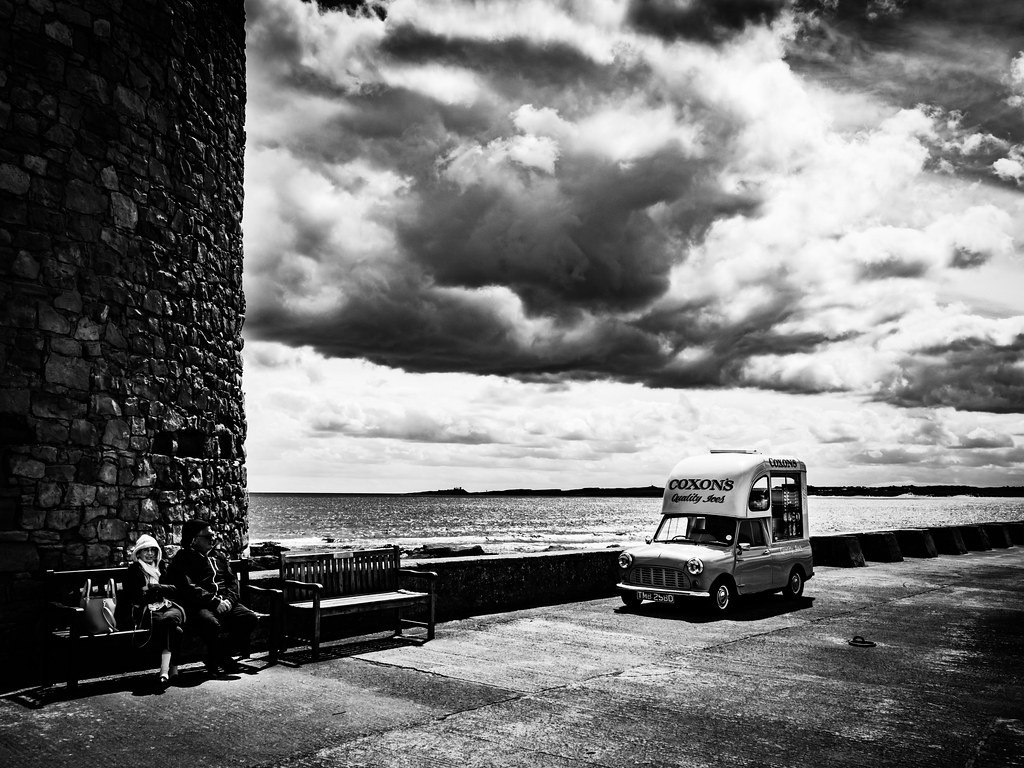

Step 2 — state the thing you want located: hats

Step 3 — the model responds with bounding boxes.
[180,519,211,547]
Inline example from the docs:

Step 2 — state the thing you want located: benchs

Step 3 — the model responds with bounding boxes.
[39,553,285,700]
[279,544,441,661]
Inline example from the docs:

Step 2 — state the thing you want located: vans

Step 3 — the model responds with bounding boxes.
[615,449,815,618]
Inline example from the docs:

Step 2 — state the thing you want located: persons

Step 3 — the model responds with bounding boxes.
[167,519,258,676]
[122,534,188,690]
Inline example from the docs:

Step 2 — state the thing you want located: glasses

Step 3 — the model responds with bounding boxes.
[199,533,216,540]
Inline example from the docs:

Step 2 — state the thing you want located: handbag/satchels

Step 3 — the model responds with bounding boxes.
[80,578,120,634]
